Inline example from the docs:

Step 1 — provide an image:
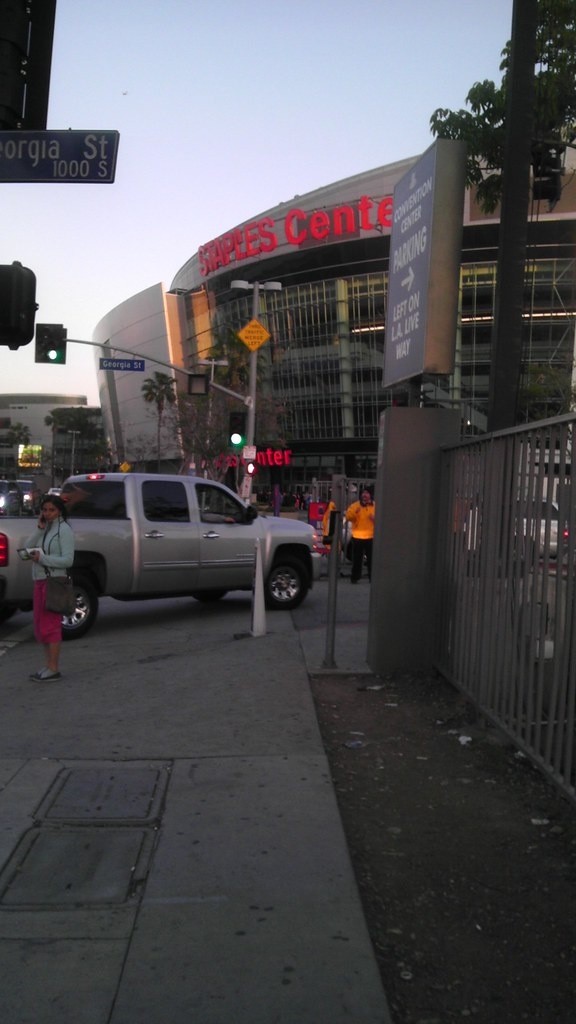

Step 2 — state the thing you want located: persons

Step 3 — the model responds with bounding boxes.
[345,487,376,584]
[281,491,312,511]
[17,494,74,683]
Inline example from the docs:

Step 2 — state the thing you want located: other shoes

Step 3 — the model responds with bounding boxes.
[351,576,358,584]
[30,666,61,683]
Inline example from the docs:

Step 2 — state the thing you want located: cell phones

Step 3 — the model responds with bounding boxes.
[41,515,46,523]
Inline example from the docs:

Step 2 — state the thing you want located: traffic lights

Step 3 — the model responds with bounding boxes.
[34,323,67,364]
[228,430,245,446]
[246,462,258,476]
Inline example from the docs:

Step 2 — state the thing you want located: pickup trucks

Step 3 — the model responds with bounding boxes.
[0,473,322,640]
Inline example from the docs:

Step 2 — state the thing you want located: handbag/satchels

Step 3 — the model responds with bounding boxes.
[45,575,75,615]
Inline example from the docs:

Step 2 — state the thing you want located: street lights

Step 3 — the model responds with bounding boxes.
[230,280,281,505]
[68,430,80,476]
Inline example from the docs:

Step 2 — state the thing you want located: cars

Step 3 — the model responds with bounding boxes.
[48,488,61,497]
[0,480,24,517]
[15,480,42,515]
